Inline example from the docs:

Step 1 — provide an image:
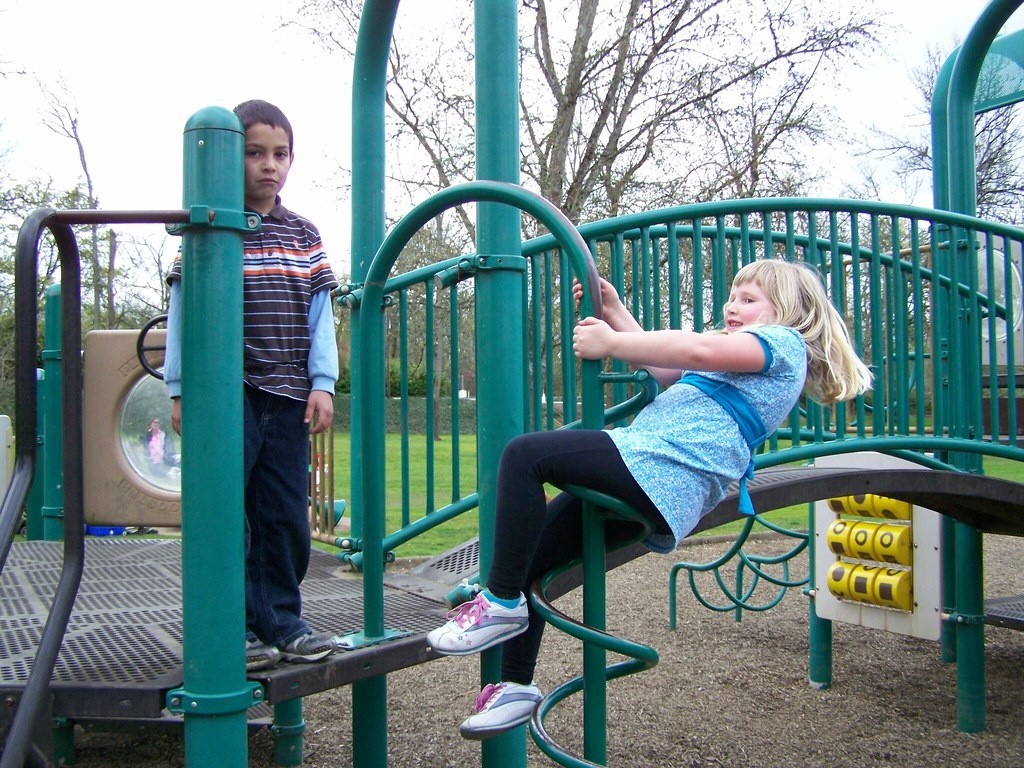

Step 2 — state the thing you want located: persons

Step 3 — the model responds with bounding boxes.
[143,418,173,468]
[427,259,874,740]
[164,100,339,672]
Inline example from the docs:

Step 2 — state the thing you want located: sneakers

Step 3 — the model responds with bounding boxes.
[460,682,544,740]
[280,633,338,664]
[246,636,280,671]
[427,588,530,656]
